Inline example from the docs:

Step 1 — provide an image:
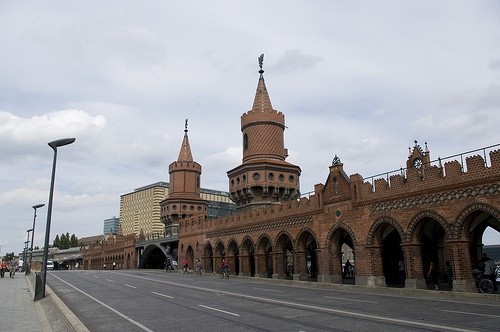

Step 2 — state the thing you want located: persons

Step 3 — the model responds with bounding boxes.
[194,258,203,276]
[59,262,69,270]
[445,260,453,290]
[112,261,116,270]
[164,257,171,273]
[182,257,189,272]
[398,257,405,286]
[0,257,24,278]
[426,261,439,290]
[344,260,355,278]
[221,259,229,276]
[479,252,497,292]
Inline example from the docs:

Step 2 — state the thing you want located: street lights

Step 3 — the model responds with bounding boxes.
[40,138,77,296]
[24,228,33,248]
[29,203,45,267]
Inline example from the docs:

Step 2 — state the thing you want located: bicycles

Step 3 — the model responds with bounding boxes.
[471,274,494,293]
[219,270,231,280]
[180,265,190,275]
[193,267,204,277]
[167,266,171,273]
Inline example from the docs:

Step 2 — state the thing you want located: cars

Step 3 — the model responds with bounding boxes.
[47,261,54,270]
